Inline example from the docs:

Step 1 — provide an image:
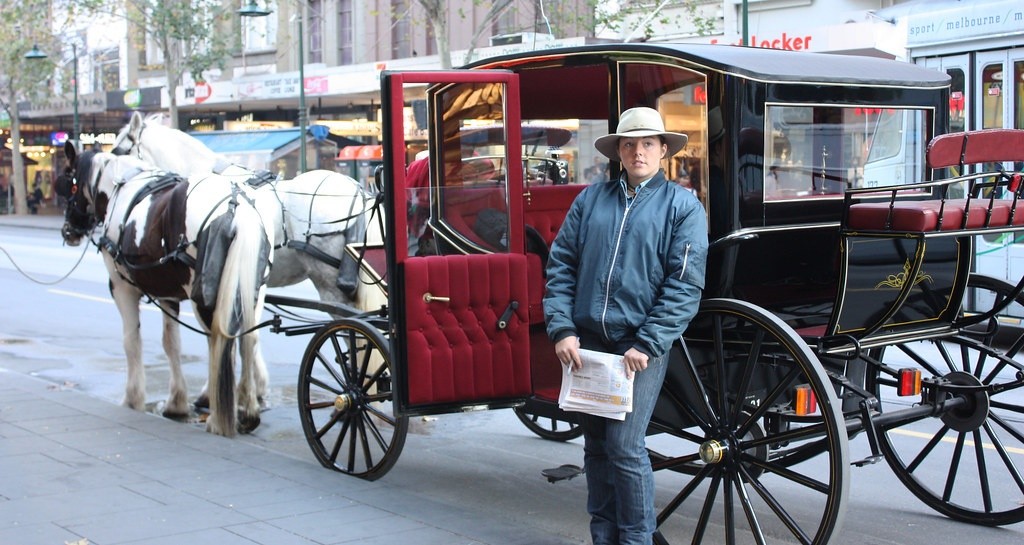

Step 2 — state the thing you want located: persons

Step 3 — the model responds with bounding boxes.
[583,156,610,184]
[405,120,496,257]
[543,107,708,545]
[28,183,42,215]
[764,132,792,199]
[0,183,14,215]
[995,160,1024,198]
[708,105,734,339]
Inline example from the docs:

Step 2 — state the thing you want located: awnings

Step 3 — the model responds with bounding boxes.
[334,144,383,162]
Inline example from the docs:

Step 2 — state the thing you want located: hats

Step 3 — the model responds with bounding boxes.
[593,107,688,161]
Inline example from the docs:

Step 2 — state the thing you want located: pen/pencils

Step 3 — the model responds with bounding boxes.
[568,336,580,374]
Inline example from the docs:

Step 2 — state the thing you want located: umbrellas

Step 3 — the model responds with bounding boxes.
[0,147,38,214]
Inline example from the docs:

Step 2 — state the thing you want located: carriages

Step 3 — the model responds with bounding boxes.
[58,44,1024,545]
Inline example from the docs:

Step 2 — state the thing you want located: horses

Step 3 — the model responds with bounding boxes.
[60,140,275,439]
[110,110,388,428]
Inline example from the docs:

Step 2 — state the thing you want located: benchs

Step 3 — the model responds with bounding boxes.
[440,180,591,328]
[843,127,1023,235]
[701,183,928,275]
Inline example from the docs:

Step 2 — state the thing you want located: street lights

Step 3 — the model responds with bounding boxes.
[238,1,307,176]
[23,40,83,155]
